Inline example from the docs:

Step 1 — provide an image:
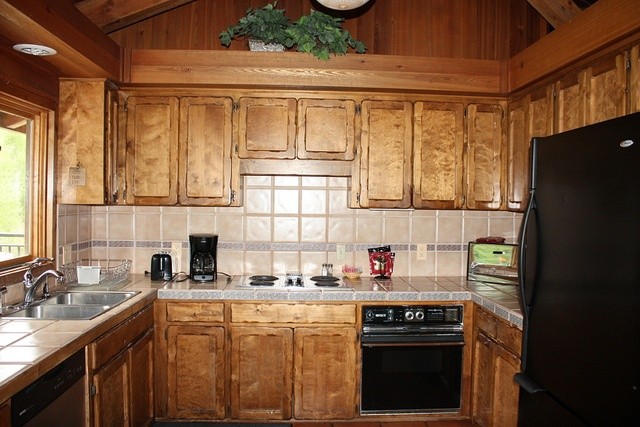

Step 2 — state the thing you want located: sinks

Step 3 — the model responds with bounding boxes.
[29,289,142,305]
[0,305,116,320]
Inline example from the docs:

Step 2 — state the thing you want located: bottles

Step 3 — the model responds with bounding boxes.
[322,264,327,277]
[328,264,333,275]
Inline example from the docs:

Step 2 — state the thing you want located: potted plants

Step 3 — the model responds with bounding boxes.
[219,3,366,61]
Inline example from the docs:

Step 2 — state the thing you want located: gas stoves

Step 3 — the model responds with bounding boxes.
[236,275,342,289]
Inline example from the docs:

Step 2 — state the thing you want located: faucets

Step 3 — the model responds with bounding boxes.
[22,261,64,303]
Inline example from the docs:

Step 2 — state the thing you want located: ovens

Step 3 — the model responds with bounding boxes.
[357,327,464,412]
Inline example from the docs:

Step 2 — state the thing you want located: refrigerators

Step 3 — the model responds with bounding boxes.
[514,113,640,424]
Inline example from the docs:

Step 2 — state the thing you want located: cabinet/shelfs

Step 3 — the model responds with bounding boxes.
[240,95,355,160]
[120,84,240,206]
[556,52,627,135]
[157,303,226,425]
[629,40,639,115]
[464,98,511,213]
[508,81,554,210]
[58,74,119,207]
[229,300,360,426]
[349,98,464,208]
[471,302,524,427]
[86,305,153,427]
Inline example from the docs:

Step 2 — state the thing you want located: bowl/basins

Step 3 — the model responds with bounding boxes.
[343,272,362,280]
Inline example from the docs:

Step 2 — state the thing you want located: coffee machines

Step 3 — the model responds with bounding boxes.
[189,235,218,282]
